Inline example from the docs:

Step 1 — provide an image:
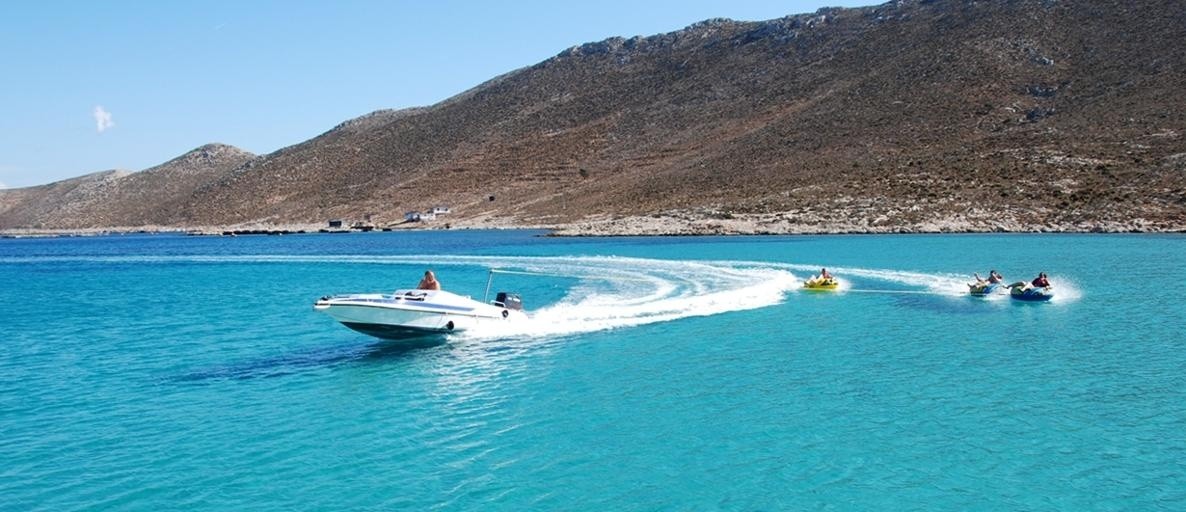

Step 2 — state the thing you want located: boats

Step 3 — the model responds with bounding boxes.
[307,286,533,348]
[800,277,843,294]
[966,276,1057,302]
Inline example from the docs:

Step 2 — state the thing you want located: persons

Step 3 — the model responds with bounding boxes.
[419,270,441,290]
[803,268,830,288]
[1003,272,1053,293]
[965,270,1003,289]
